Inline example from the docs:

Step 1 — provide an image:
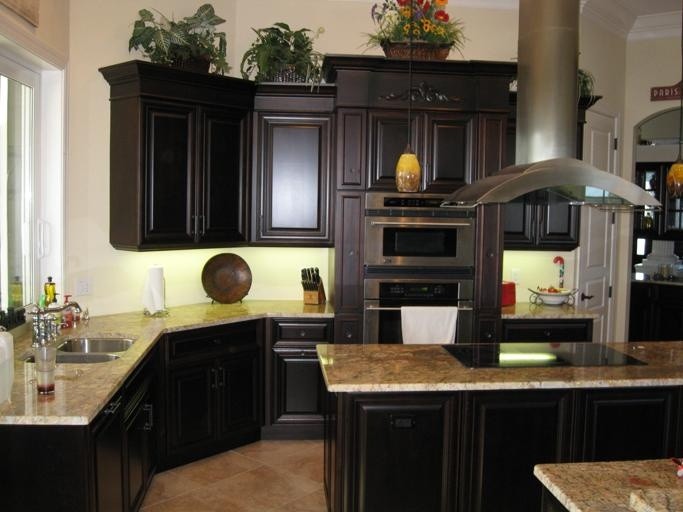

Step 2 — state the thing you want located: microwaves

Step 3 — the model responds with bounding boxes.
[364,192,475,270]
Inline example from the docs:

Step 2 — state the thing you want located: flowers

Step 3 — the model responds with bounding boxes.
[357,0,471,59]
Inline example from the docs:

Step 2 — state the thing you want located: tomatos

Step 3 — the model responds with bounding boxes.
[548,286,557,292]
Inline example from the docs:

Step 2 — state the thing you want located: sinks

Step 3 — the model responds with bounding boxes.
[16,353,119,364]
[53,336,137,353]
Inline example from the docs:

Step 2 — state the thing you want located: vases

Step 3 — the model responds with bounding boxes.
[380,41,455,63]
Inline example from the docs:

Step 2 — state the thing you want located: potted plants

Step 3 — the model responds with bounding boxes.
[128,4,233,76]
[239,19,328,91]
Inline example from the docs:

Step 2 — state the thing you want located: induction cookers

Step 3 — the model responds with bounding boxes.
[441,342,649,368]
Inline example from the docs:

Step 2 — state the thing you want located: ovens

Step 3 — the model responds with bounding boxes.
[364,272,474,343]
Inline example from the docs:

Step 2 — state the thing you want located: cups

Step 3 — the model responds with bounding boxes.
[34,347,57,395]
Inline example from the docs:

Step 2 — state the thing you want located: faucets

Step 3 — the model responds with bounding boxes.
[26,299,85,317]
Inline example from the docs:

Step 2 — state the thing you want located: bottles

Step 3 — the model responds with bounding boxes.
[650,174,656,189]
[644,212,653,228]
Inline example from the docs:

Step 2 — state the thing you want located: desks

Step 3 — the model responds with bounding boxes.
[533,458,683,512]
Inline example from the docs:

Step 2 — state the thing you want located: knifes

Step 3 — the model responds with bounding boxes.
[301,267,320,291]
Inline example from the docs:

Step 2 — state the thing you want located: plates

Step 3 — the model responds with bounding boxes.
[201,253,252,304]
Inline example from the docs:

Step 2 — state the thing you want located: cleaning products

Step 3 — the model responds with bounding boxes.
[62,293,74,326]
[9,275,23,307]
[46,292,63,326]
[43,275,56,304]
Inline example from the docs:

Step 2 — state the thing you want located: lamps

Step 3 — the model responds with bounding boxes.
[395,0,421,192]
[666,89,683,196]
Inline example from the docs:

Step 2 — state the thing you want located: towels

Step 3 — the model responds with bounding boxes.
[400,306,459,344]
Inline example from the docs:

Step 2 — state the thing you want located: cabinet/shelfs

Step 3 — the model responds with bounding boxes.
[98,58,253,252]
[480,61,602,250]
[463,382,683,512]
[163,317,265,471]
[321,52,517,192]
[250,88,336,248]
[121,337,163,512]
[630,272,683,341]
[265,316,332,440]
[0,389,122,512]
[323,381,464,512]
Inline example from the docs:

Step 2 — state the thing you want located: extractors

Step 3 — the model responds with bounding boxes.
[439,0,663,214]
